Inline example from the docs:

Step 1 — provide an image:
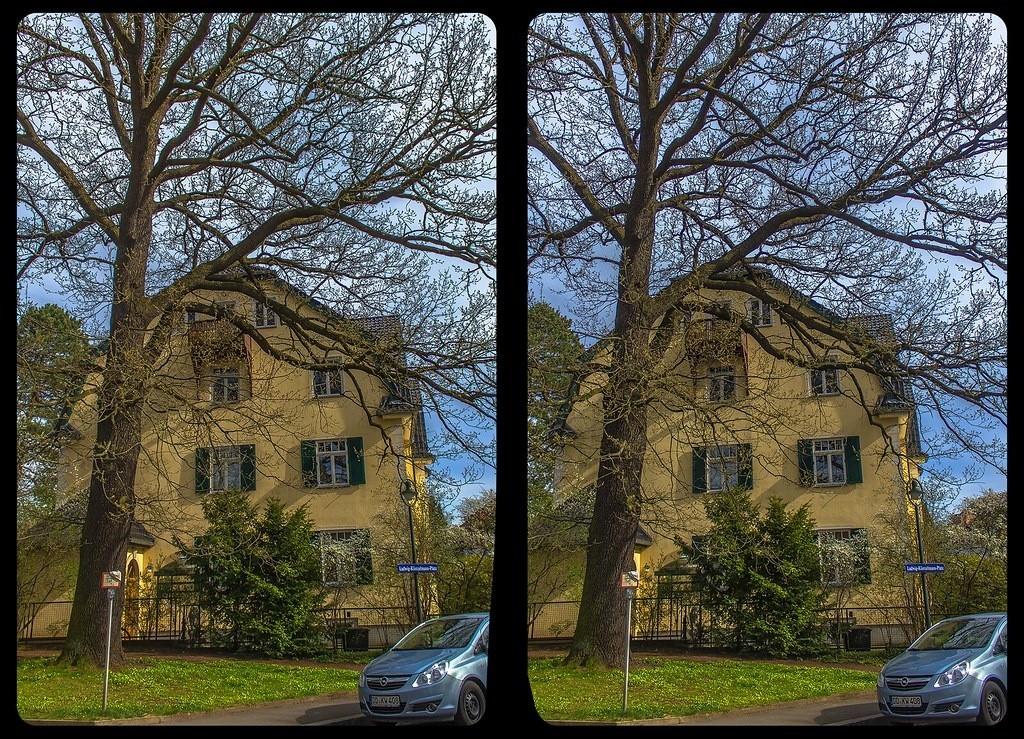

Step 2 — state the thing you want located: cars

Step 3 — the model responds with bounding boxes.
[359,612,490,727]
[877,612,1008,726]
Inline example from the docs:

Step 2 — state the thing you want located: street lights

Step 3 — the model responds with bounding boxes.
[400,478,422,626]
[906,478,932,630]
